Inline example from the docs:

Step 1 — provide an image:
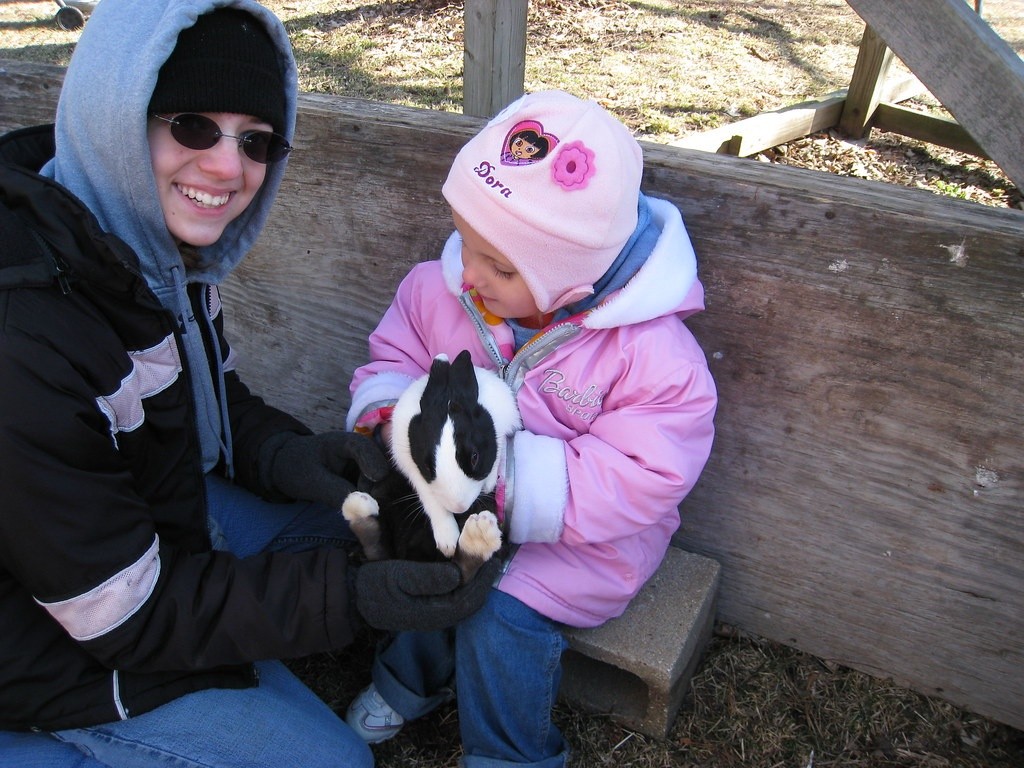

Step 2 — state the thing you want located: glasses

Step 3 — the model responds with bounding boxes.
[150,114,293,164]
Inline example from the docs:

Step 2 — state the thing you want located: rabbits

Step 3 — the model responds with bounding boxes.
[342,350,523,584]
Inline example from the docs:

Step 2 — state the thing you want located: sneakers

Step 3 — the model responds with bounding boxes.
[347,681,405,744]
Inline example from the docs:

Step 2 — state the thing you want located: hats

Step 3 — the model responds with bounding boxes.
[150,6,288,114]
[441,89,643,316]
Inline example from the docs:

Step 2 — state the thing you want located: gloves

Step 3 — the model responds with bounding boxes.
[388,351,522,556]
[262,432,388,510]
[348,557,500,634]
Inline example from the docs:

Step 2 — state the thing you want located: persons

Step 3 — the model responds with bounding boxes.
[343,88,719,768]
[0,0,505,768]
